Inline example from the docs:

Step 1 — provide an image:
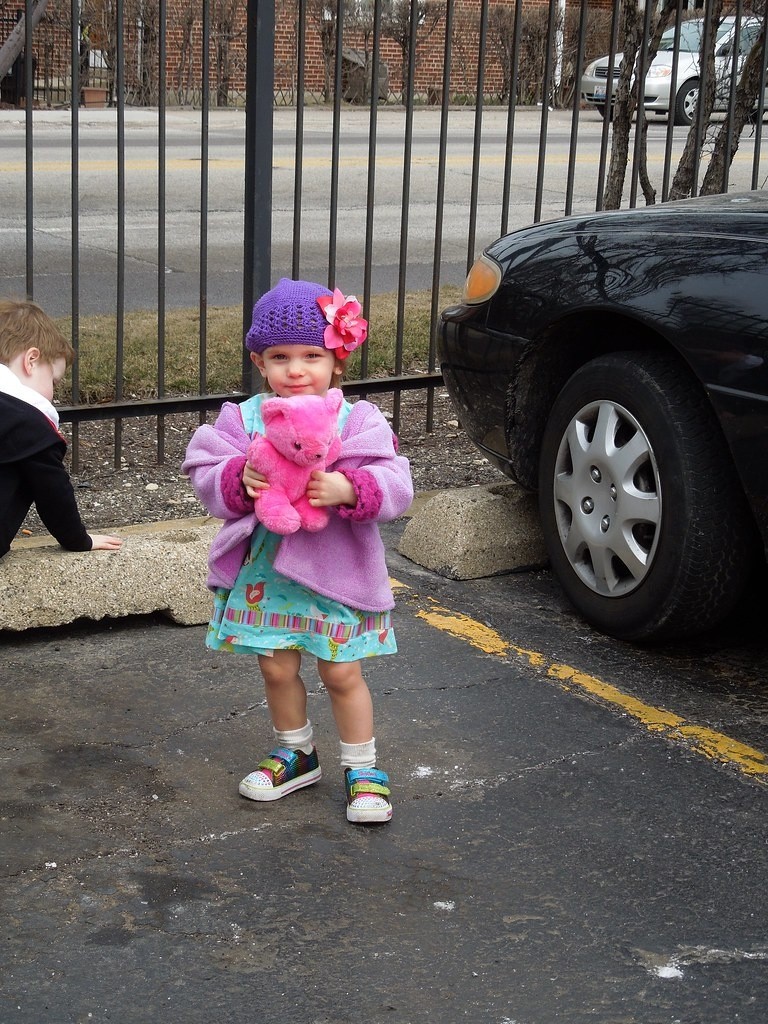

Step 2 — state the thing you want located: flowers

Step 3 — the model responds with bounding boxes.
[317,286,368,360]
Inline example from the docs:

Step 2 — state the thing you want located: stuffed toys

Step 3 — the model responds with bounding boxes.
[246,388,343,536]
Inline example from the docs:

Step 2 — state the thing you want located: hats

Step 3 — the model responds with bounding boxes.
[245,277,367,360]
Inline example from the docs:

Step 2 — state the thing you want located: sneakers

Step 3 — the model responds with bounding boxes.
[238,746,324,802]
[344,767,393,823]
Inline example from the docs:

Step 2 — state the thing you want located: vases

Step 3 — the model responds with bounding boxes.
[79,87,110,108]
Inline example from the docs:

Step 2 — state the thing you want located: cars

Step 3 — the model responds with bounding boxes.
[436,189,768,649]
[581,16,768,126]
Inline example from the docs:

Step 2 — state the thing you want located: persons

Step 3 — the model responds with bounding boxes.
[181,277,414,822]
[0,299,122,558]
